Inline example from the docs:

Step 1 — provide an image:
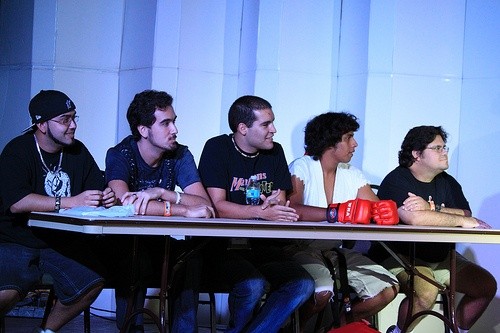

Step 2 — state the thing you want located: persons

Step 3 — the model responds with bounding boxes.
[106,90,216,333]
[0,90,117,333]
[376,125,497,333]
[288,111,399,333]
[198,93,316,333]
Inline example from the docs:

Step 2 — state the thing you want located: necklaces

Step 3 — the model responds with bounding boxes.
[33,134,63,194]
[232,134,259,158]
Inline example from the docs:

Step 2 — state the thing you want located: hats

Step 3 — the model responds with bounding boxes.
[21,90,76,132]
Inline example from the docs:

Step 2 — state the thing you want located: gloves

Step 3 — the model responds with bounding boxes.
[326,198,374,224]
[371,199,399,225]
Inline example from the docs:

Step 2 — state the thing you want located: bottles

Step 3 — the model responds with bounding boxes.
[246,175,261,219]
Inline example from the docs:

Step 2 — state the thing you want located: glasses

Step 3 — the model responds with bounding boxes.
[51,115,80,125]
[421,145,450,154]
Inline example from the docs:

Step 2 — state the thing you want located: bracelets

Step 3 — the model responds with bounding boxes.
[429,202,441,212]
[55,197,61,212]
[175,191,182,204]
[163,200,171,216]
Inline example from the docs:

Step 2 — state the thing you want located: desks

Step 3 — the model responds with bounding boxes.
[26,211,500,333]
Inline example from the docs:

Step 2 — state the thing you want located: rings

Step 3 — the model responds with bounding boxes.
[133,194,137,196]
